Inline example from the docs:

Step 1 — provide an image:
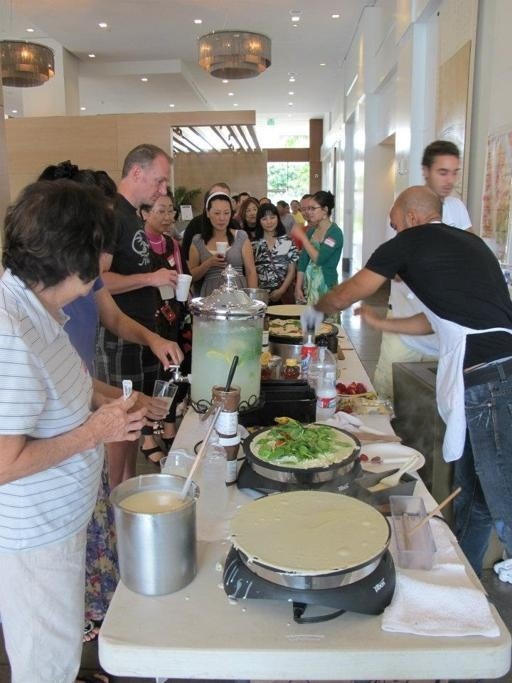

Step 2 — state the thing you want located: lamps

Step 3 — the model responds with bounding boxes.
[0,40,54,87]
[199,31,271,79]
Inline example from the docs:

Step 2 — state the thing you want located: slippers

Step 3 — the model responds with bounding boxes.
[161,434,174,447]
[142,445,166,466]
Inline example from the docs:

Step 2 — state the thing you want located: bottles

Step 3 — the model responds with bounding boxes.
[261,324,338,419]
[219,433,242,487]
[212,384,241,437]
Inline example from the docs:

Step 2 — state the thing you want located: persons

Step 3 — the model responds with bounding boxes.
[181,181,231,262]
[140,185,194,468]
[313,184,512,581]
[231,188,344,325]
[188,191,258,300]
[33,157,184,645]
[372,140,473,400]
[0,177,151,683]
[95,142,179,490]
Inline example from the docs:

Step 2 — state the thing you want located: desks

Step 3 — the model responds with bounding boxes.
[98,321,512,683]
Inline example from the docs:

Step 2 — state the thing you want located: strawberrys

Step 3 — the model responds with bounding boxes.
[336,379,368,395]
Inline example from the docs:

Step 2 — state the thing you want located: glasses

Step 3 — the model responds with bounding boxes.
[308,207,322,211]
[152,208,178,217]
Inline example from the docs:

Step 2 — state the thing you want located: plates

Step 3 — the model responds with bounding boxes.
[358,442,426,474]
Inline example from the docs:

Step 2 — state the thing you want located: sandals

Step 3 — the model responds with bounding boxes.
[82,620,100,643]
[78,674,109,683]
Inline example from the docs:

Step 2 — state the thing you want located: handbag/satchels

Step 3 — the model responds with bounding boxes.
[279,280,296,303]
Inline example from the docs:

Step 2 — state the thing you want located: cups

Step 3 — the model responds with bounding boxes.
[175,274,193,302]
[152,380,178,410]
[109,473,200,597]
[216,241,228,258]
[295,220,309,250]
[160,454,188,478]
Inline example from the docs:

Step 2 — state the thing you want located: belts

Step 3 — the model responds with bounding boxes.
[463,358,511,389]
